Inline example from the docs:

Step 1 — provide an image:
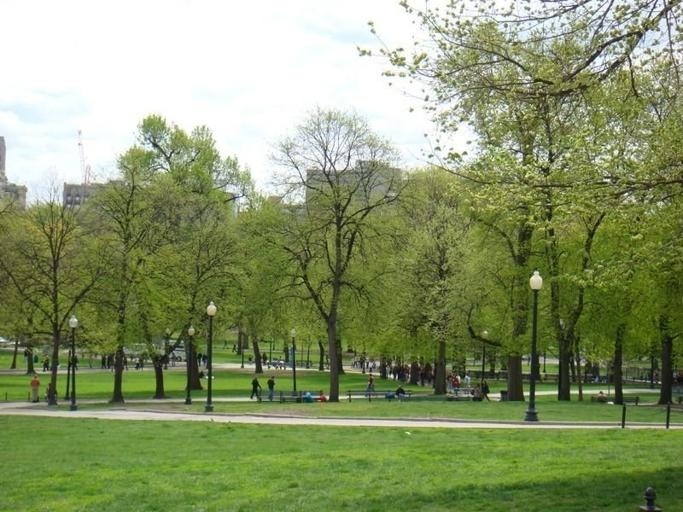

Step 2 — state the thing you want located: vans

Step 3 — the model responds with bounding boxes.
[160,340,186,361]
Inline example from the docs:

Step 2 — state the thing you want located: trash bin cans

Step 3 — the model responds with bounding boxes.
[501,391,507,400]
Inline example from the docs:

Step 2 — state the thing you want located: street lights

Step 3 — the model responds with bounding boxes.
[202,300,216,412]
[478,330,489,380]
[68,315,79,411]
[163,328,169,369]
[523,271,544,422]
[290,328,297,391]
[183,325,196,404]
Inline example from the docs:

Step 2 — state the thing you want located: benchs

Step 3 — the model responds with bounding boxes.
[447,388,478,401]
[591,396,639,406]
[256,391,324,403]
[345,390,412,402]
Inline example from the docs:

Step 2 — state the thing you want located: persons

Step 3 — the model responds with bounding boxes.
[29,375,41,403]
[44,383,58,401]
[597,390,607,402]
[201,354,208,368]
[319,390,326,402]
[302,390,313,402]
[247,354,253,365]
[265,375,276,400]
[21,347,176,372]
[351,356,491,402]
[262,351,268,365]
[231,343,238,353]
[249,376,262,399]
[196,351,202,366]
